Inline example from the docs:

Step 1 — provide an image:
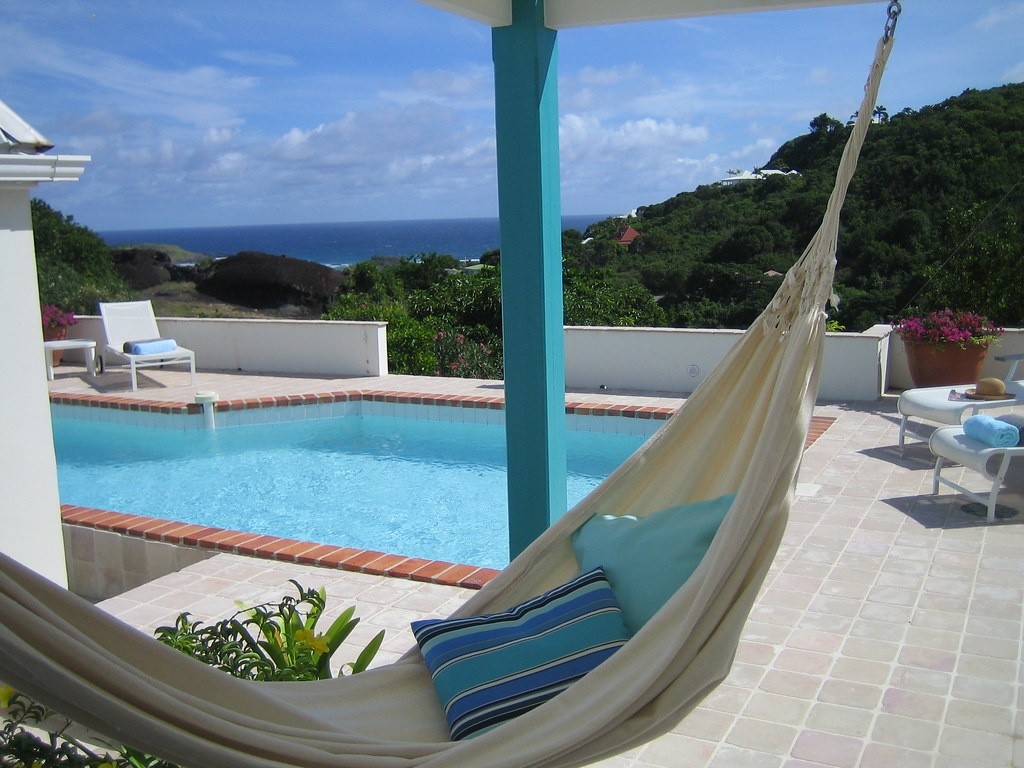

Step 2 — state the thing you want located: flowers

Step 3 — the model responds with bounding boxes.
[40,304,80,331]
[890,309,1005,351]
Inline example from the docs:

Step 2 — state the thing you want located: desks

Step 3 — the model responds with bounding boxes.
[44,339,96,381]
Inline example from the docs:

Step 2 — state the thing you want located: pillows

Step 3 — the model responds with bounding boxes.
[572,492,737,638]
[123,338,178,355]
[402,563,633,743]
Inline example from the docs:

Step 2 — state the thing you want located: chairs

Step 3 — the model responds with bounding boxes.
[896,354,1024,461]
[99,300,196,392]
[928,425,1024,525]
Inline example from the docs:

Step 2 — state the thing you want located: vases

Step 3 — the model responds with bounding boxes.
[903,341,991,388]
[43,326,67,367]
